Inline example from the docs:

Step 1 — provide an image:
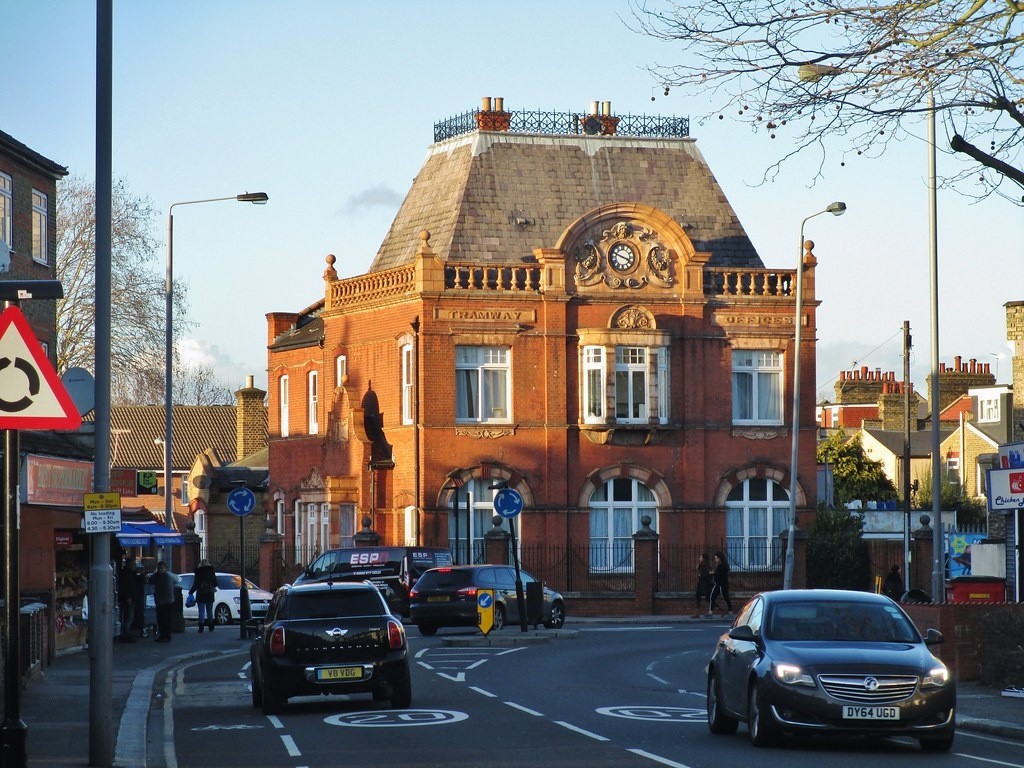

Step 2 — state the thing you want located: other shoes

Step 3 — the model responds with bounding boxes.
[154,637,170,643]
[705,613,713,619]
[725,615,734,619]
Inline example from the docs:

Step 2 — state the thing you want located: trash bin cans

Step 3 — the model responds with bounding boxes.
[526,581,545,630]
[171,585,183,632]
[945,576,1006,603]
[900,589,933,604]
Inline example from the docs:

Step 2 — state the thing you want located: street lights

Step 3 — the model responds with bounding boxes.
[782,201,848,590]
[800,61,946,604]
[163,192,269,572]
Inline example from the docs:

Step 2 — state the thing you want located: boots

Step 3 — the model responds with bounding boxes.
[691,609,699,618]
[717,606,728,618]
[197,623,205,633]
[208,622,215,632]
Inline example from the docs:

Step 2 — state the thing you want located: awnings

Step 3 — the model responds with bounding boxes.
[114,524,152,547]
[128,523,185,546]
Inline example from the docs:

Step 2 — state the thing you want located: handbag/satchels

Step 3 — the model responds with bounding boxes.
[185,594,196,608]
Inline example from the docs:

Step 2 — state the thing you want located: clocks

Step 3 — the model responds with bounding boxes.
[608,239,634,270]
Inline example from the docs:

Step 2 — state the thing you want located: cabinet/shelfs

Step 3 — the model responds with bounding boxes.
[55,569,89,649]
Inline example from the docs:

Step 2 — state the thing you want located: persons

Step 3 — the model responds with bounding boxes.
[690,551,733,618]
[117,558,174,642]
[189,559,218,633]
[882,566,903,601]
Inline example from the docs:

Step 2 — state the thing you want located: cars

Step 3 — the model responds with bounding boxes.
[706,589,956,752]
[146,573,274,625]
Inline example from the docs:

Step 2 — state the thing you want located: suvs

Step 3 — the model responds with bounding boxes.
[249,579,411,714]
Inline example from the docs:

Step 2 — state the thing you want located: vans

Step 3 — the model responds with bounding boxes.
[291,545,454,619]
[410,563,566,635]
